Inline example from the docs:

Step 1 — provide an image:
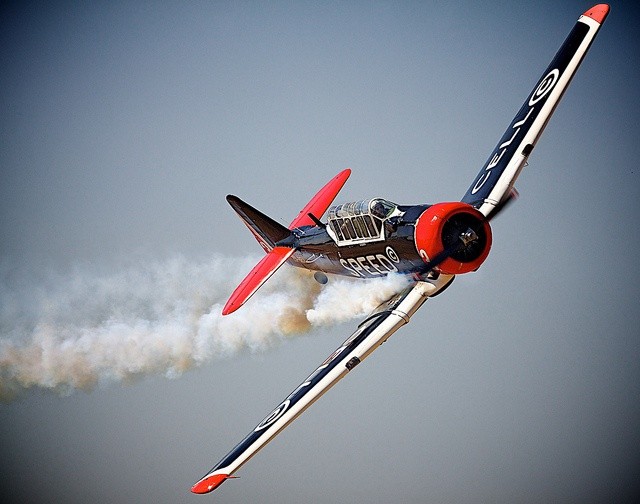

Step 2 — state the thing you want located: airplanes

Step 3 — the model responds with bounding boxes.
[177,3,613,495]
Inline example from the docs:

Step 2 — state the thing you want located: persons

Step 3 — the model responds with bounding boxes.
[371,200,390,218]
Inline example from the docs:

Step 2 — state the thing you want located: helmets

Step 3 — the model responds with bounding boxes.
[370,200,381,209]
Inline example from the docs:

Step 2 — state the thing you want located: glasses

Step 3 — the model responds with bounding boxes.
[377,206,383,209]
